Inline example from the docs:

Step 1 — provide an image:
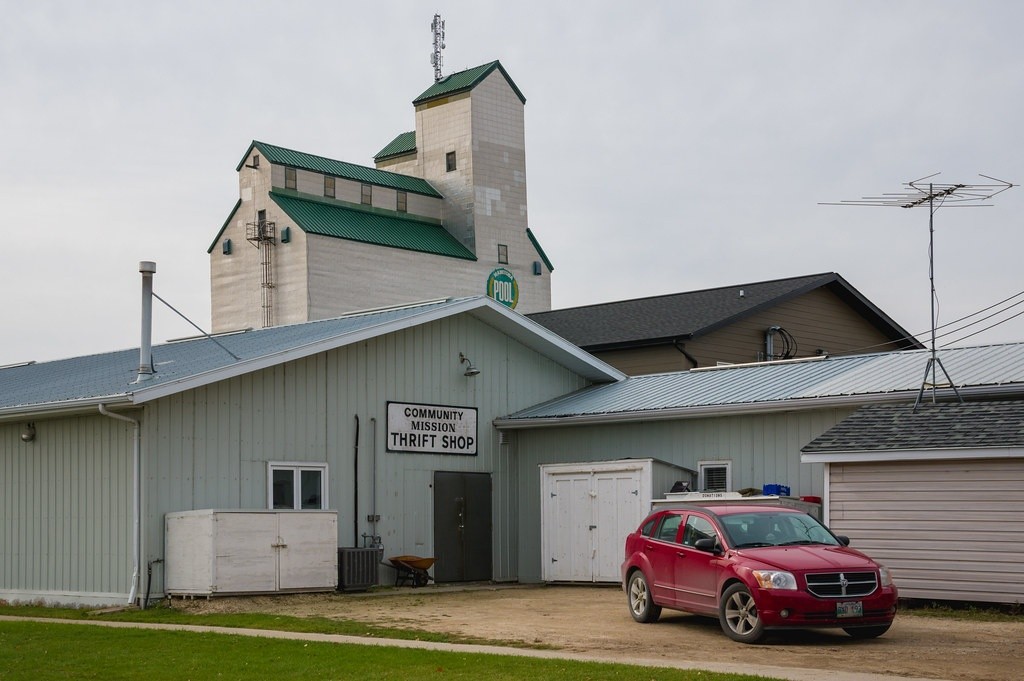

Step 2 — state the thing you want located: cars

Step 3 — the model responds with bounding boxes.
[619,504,900,643]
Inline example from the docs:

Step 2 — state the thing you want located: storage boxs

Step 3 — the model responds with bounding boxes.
[164,509,340,600]
[762,483,790,497]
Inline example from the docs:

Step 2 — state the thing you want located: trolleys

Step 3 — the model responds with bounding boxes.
[380,554,436,588]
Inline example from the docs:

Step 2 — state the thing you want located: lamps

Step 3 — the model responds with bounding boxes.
[461,357,481,377]
[670,481,691,493]
